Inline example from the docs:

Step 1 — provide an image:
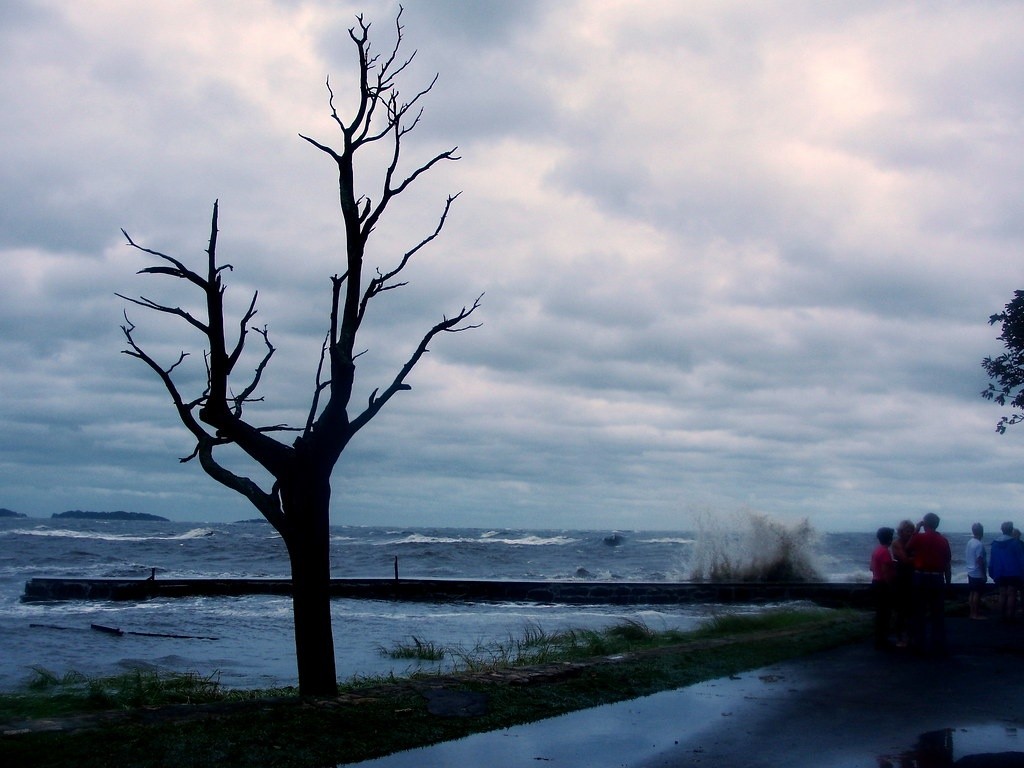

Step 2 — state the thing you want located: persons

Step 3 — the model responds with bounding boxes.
[988,522,1024,624]
[965,523,987,619]
[904,514,951,657]
[892,521,915,646]
[1012,529,1024,547]
[872,528,898,652]
